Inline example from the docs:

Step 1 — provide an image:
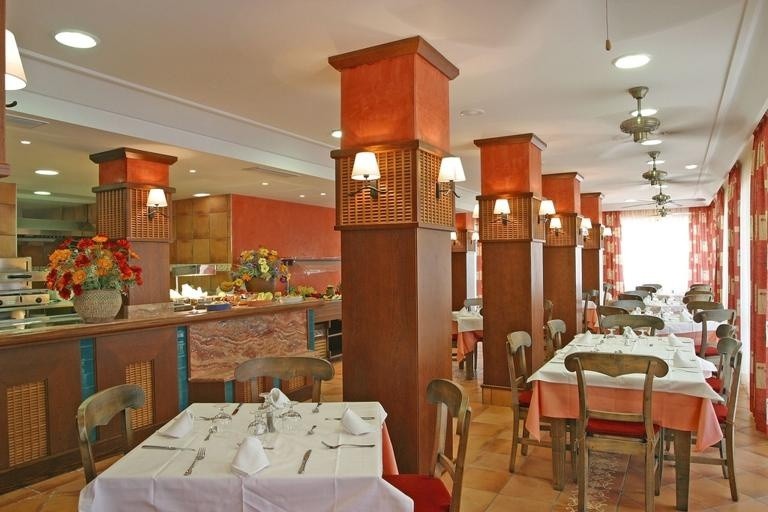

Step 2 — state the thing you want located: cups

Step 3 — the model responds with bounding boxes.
[470,305,476,312]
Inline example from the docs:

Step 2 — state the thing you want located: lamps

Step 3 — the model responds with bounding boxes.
[4,30,27,107]
[347,149,612,244]
[143,188,171,221]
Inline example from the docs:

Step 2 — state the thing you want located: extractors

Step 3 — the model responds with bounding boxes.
[16,218,96,245]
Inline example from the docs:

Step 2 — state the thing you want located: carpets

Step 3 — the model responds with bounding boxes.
[548,450,631,512]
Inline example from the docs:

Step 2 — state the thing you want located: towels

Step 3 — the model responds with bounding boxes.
[157,387,367,476]
[459,306,468,314]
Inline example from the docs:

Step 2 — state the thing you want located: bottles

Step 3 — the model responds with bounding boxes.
[325,285,336,296]
[249,400,304,437]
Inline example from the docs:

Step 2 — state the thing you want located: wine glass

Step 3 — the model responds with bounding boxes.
[210,401,234,435]
[605,328,617,344]
[637,329,648,346]
[633,290,676,321]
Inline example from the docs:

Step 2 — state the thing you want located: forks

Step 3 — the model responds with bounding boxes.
[185,446,209,477]
[309,423,315,435]
[320,440,376,451]
[311,401,321,413]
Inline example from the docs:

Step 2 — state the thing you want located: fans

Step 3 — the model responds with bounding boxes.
[611,85,707,217]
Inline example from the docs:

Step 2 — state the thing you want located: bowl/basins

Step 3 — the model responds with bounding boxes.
[176,291,283,311]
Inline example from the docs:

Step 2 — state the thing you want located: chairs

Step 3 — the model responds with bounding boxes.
[463,298,483,309]
[505,331,578,486]
[233,356,335,403]
[543,282,665,359]
[564,352,668,512]
[76,382,145,484]
[662,283,743,502]
[382,379,472,512]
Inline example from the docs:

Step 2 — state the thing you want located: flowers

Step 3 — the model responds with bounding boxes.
[231,245,291,286]
[44,233,144,298]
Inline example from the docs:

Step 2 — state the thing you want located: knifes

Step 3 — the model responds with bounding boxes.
[323,417,374,422]
[297,448,313,476]
[141,445,194,453]
[232,401,246,415]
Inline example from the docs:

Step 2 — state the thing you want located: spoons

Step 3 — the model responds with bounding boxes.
[203,425,217,441]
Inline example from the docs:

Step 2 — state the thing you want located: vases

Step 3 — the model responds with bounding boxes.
[245,276,275,296]
[73,288,123,324]
